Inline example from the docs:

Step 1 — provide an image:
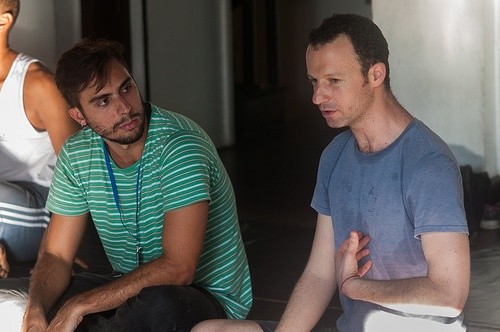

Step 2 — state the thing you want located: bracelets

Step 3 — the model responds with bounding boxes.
[340,274,360,292]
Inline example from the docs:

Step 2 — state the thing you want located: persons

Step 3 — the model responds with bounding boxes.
[190,12,470,332]
[0,1,82,279]
[22,35,253,332]
